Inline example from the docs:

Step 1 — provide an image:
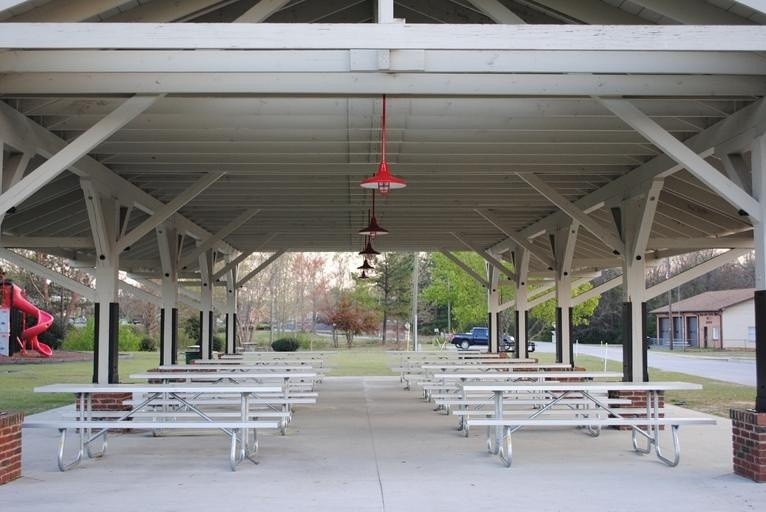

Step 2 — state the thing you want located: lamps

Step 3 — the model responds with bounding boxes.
[357,256,370,281]
[356,235,375,270]
[359,94,409,197]
[355,172,390,241]
[357,209,381,259]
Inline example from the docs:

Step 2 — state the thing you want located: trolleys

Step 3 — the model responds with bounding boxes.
[498,335,537,353]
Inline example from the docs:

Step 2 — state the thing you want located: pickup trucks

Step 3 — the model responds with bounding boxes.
[450,326,514,352]
